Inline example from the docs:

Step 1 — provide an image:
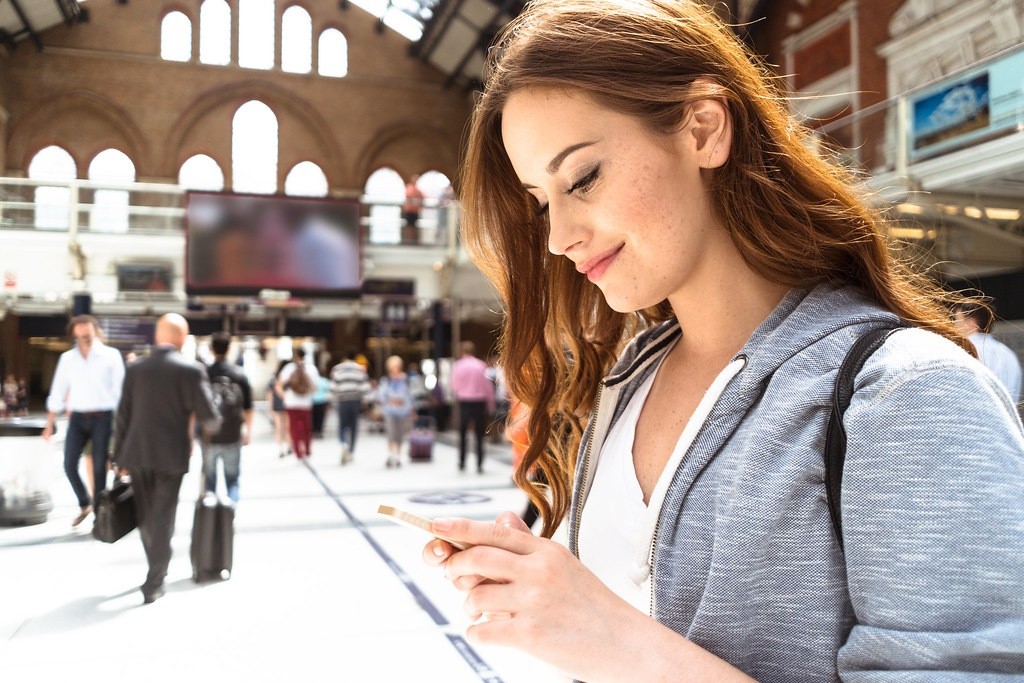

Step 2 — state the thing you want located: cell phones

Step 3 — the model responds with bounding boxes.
[377,505,473,553]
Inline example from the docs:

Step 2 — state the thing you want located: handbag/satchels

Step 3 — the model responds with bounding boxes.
[92,474,137,543]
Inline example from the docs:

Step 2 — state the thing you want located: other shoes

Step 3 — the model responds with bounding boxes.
[386,456,400,467]
[341,444,349,465]
[145,580,167,603]
[73,505,91,527]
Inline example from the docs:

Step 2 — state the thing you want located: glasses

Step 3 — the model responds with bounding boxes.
[73,334,92,343]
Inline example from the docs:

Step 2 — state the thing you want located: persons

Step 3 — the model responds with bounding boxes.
[951,295,1023,405]
[0,330,510,503]
[111,313,223,604]
[421,0,1024,682]
[400,172,424,228]
[42,313,126,527]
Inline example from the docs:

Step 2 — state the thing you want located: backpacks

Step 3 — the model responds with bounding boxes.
[289,361,309,393]
[197,368,243,442]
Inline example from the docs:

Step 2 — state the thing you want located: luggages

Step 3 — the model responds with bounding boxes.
[409,429,433,460]
[190,489,235,582]
